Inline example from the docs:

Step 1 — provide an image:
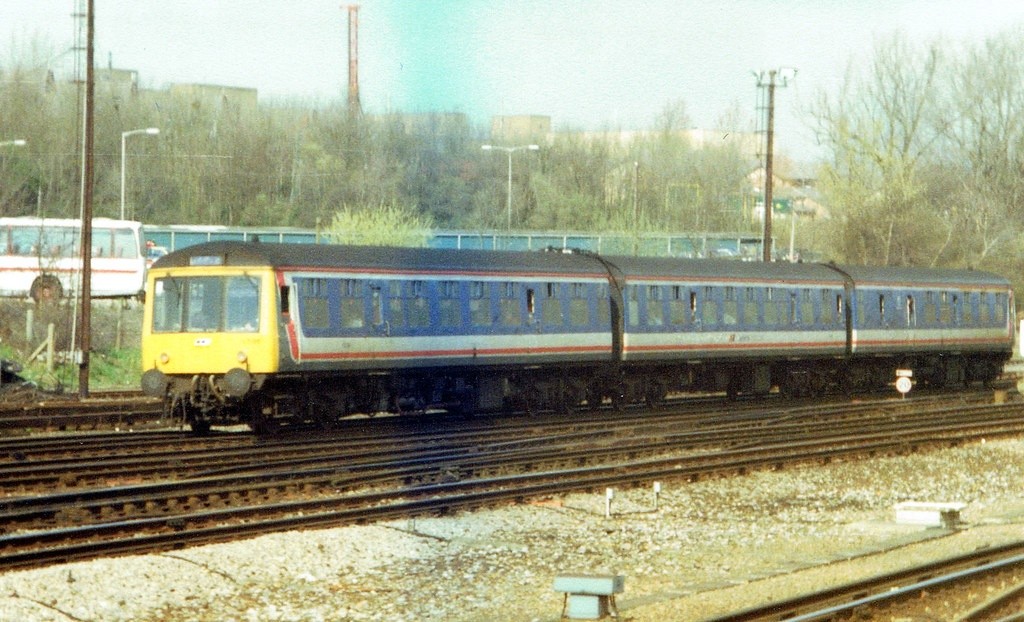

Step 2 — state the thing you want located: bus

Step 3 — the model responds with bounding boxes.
[1,214,143,305]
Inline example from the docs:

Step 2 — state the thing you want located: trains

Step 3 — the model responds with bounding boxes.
[141,220,777,262]
[129,238,1018,437]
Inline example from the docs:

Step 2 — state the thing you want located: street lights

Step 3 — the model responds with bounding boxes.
[117,126,160,220]
[479,144,541,229]
